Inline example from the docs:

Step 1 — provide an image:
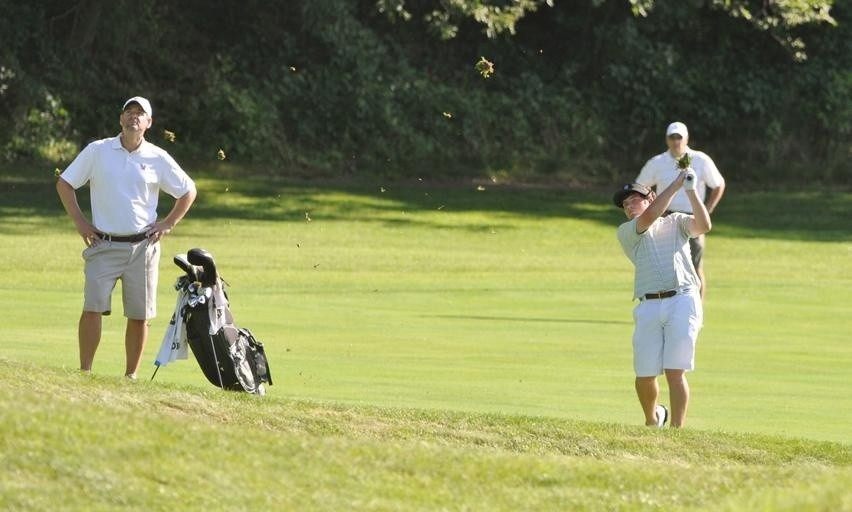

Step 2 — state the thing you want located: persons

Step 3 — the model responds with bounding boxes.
[611,168,714,431]
[635,122,727,298]
[56,95,197,382]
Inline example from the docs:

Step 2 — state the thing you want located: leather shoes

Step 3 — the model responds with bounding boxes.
[684,168,698,191]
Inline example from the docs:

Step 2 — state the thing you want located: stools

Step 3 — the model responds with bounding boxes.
[638,289,676,301]
[92,231,157,242]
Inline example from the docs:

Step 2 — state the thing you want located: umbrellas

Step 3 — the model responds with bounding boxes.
[646,405,670,429]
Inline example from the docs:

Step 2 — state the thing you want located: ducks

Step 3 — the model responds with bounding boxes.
[176,249,275,394]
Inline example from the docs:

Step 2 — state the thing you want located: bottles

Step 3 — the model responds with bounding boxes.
[667,121,688,141]
[611,182,652,207]
[121,94,154,122]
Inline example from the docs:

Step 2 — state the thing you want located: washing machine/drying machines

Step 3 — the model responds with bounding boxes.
[173,248,215,310]
[679,153,692,182]
